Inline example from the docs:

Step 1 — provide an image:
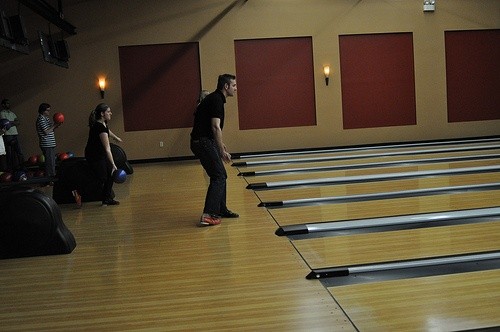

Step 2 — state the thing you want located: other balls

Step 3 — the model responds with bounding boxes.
[53,112,65,123]
[28,150,75,164]
[113,169,127,185]
[1,173,12,182]
[0,118,10,131]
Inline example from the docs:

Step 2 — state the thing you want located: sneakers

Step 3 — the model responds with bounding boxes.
[200,215,221,225]
[102,199,120,206]
[215,209,239,218]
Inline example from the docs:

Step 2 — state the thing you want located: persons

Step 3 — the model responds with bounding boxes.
[190,74,239,225]
[84,103,122,205]
[0,99,26,170]
[35,103,59,176]
